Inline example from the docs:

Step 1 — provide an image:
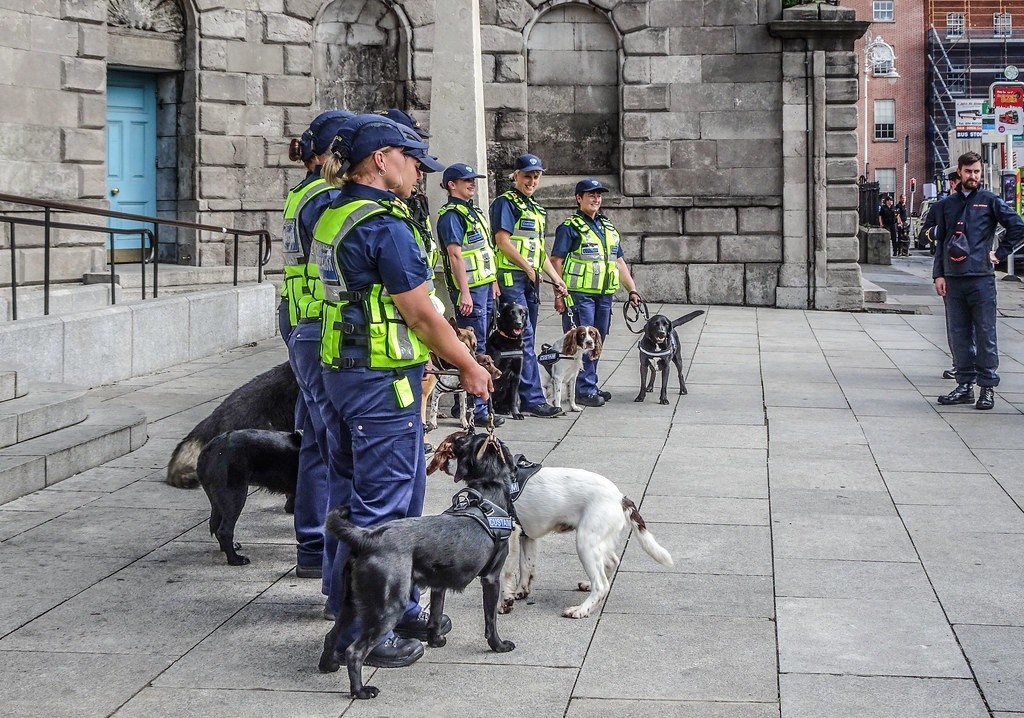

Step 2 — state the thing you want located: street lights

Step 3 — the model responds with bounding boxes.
[863,28,903,180]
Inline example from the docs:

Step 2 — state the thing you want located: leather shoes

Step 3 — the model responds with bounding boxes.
[938,385,975,404]
[943,369,957,380]
[976,387,994,409]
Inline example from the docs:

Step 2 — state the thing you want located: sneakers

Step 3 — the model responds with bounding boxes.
[363,632,425,667]
[425,443,434,461]
[576,391,605,407]
[519,402,564,418]
[596,389,611,401]
[474,413,504,426]
[392,611,452,640]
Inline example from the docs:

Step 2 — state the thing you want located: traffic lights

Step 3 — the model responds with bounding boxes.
[910,178,916,193]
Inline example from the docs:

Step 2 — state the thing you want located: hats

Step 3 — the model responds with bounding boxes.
[575,180,609,195]
[331,108,446,172]
[943,165,960,180]
[442,163,486,187]
[301,111,357,164]
[886,196,894,201]
[515,154,544,172]
[946,232,972,274]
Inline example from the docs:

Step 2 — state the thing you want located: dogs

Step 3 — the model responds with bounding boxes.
[316,432,675,697]
[535,325,602,413]
[167,360,304,565]
[418,317,502,431]
[633,310,705,405]
[485,302,528,420]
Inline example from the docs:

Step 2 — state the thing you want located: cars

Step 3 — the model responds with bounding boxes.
[909,199,937,250]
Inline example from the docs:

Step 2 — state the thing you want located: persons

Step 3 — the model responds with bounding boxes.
[896,194,907,256]
[879,196,900,256]
[437,164,505,428]
[490,154,568,418]
[551,179,641,407]
[276,106,494,669]
[918,166,979,383]
[932,152,1024,409]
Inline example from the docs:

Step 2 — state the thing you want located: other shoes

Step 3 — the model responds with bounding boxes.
[296,564,323,579]
[324,603,334,621]
[893,252,897,255]
